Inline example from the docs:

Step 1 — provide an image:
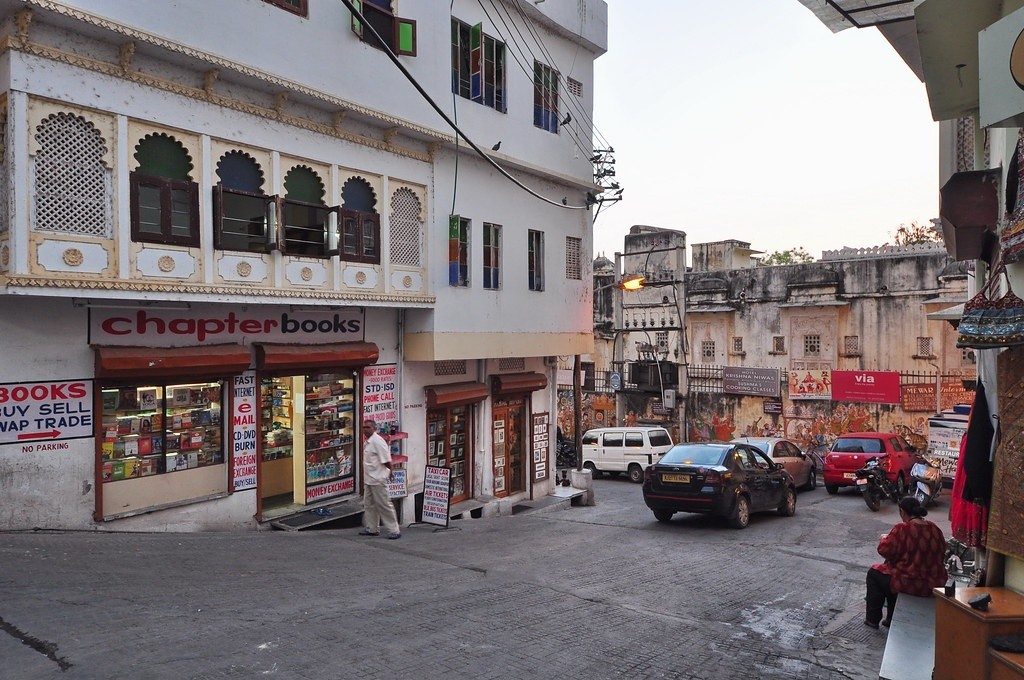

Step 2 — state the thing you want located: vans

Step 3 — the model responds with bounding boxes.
[579,426,674,483]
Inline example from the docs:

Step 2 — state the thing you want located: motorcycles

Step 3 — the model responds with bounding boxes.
[855,453,897,511]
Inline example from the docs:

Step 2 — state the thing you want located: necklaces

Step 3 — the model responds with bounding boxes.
[908,516,922,522]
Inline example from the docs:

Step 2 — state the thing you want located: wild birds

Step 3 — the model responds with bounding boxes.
[492,141,502,151]
[560,112,572,126]
[562,154,624,210]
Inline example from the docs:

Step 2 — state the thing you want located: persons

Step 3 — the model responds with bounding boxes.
[359,420,401,539]
[865,497,948,629]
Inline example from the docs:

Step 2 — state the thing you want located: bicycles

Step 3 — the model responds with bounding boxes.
[805,433,825,466]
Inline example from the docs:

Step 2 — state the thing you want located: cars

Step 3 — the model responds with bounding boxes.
[823,431,920,494]
[642,442,797,528]
[729,436,818,491]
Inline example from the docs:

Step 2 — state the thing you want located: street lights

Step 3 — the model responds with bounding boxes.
[572,276,647,472]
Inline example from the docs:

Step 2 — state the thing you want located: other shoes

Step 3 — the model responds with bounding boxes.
[359,531,379,536]
[388,534,401,539]
[864,620,879,629]
[882,619,890,627]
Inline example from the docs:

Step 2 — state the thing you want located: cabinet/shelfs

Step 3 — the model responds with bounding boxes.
[100,400,223,486]
[260,377,355,506]
[930,585,1024,680]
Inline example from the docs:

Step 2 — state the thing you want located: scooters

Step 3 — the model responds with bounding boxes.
[909,448,947,510]
